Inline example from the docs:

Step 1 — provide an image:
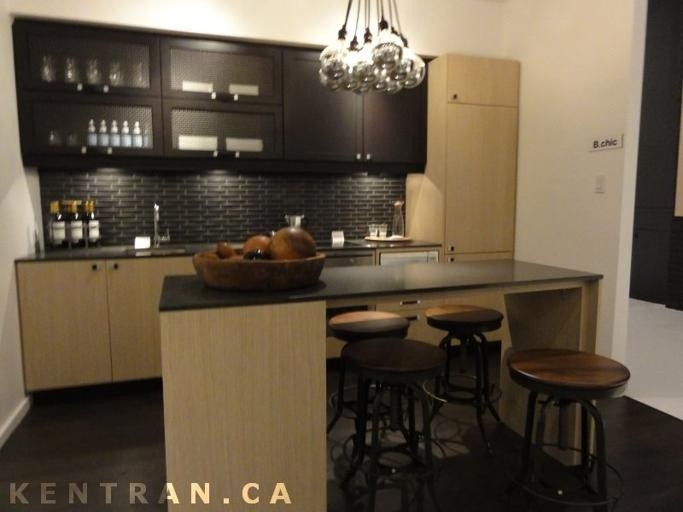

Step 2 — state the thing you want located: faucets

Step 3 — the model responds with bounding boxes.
[154,204,170,247]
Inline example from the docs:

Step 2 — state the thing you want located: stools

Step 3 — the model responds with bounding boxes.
[507,349,630,512]
[327,310,409,444]
[422,304,503,457]
[342,338,447,512]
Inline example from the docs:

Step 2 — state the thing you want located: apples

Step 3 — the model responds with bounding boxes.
[242,234,270,255]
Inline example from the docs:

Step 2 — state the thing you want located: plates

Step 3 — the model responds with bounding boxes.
[191,248,327,289]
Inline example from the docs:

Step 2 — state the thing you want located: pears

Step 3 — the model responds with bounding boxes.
[217,241,237,258]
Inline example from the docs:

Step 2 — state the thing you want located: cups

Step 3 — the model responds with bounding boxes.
[87,57,106,85]
[109,59,126,86]
[63,57,79,83]
[39,56,56,82]
[129,61,145,87]
[378,224,388,238]
[367,224,378,238]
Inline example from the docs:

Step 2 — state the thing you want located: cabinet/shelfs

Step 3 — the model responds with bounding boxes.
[160,31,283,172]
[283,45,427,173]
[406,56,520,346]
[16,244,197,394]
[318,248,376,267]
[13,18,163,169]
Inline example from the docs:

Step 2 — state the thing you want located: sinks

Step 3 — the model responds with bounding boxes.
[125,248,187,257]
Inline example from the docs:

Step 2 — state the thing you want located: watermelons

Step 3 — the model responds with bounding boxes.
[269,227,316,261]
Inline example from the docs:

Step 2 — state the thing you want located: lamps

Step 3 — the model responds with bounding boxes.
[318,0,426,94]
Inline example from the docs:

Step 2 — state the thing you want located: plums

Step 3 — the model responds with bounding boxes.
[243,248,270,260]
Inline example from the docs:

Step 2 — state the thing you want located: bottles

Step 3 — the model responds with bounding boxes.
[110,120,120,147]
[120,120,132,148]
[87,119,98,147]
[392,200,405,238]
[98,120,109,147]
[46,199,100,249]
[132,121,142,148]
